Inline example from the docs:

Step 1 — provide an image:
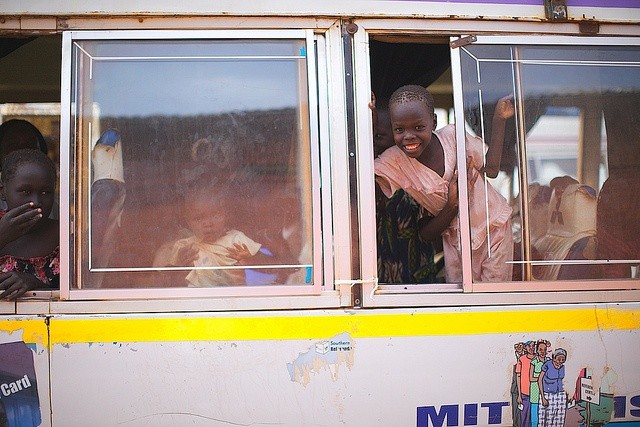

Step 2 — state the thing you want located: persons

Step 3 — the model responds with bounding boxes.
[0,148,59,301]
[0,119,49,165]
[373,95,480,283]
[173,219,302,285]
[368,84,515,283]
[160,184,288,288]
[510,340,567,423]
[551,176,578,224]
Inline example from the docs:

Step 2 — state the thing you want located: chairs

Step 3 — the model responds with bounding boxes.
[513,182,551,281]
[534,185,600,279]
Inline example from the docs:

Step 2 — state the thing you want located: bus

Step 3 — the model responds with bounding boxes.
[0,0,640,427]
